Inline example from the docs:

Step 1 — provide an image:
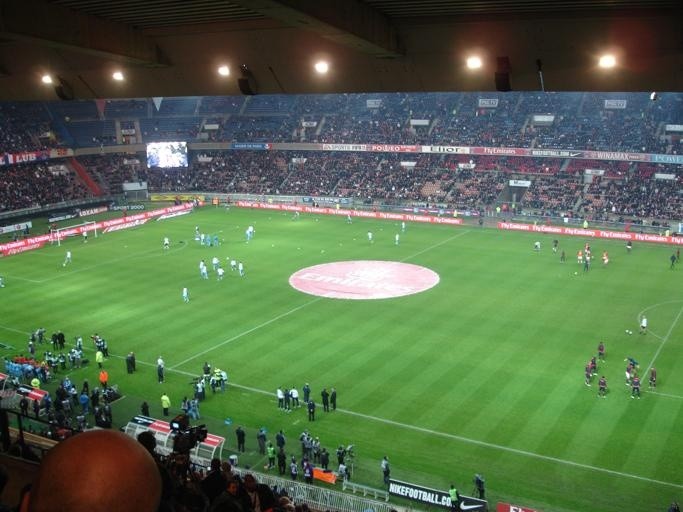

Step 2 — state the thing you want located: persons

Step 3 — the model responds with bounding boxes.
[182,286,190,303]
[626,238,632,252]
[676,247,680,258]
[62,251,71,267]
[533,239,541,255]
[194,228,224,247]
[667,501,680,512]
[395,232,399,245]
[574,241,609,272]
[1,91,682,229]
[583,339,656,400]
[560,249,564,263]
[380,455,390,483]
[448,485,459,512]
[639,314,647,334]
[551,238,558,255]
[199,255,244,281]
[244,226,252,243]
[367,230,373,242]
[163,237,168,248]
[669,254,676,269]
[2,327,351,512]
